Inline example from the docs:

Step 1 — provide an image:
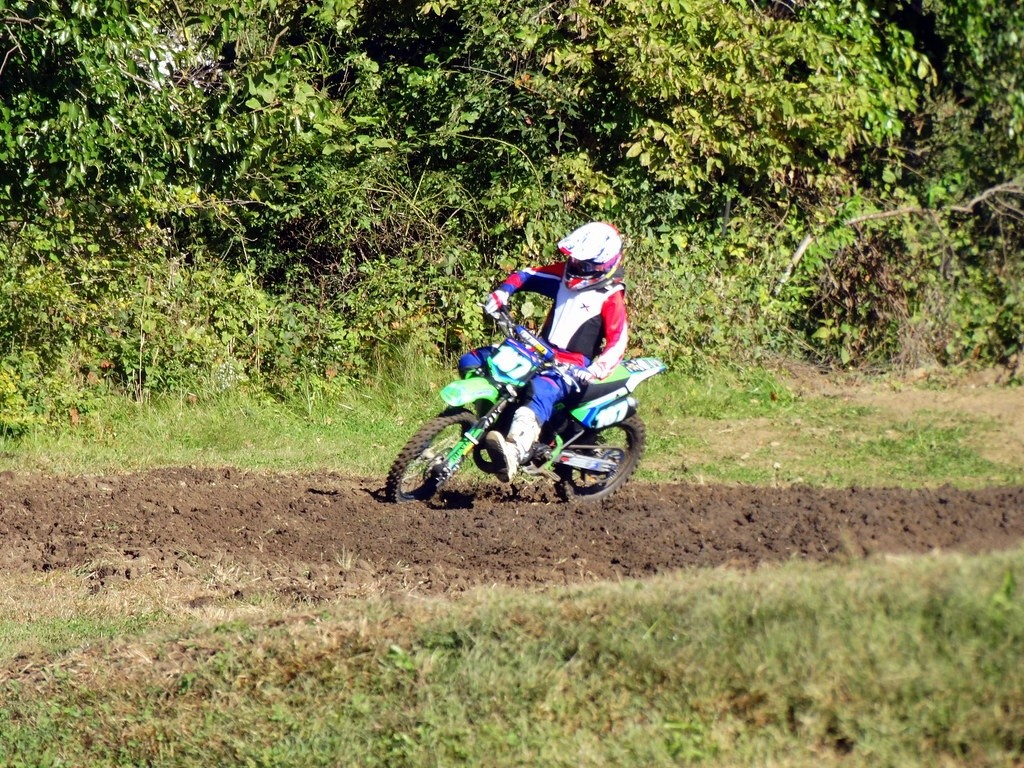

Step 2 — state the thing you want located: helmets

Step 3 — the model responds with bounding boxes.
[558,221,624,291]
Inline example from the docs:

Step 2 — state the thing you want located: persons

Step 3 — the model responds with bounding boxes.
[483,222,627,482]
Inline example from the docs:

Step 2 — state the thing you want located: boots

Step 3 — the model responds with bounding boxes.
[486,406,541,485]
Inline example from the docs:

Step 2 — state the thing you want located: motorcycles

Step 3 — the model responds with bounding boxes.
[384,305,668,505]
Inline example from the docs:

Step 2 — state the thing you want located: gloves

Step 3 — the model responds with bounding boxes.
[485,290,509,314]
[573,363,600,380]
